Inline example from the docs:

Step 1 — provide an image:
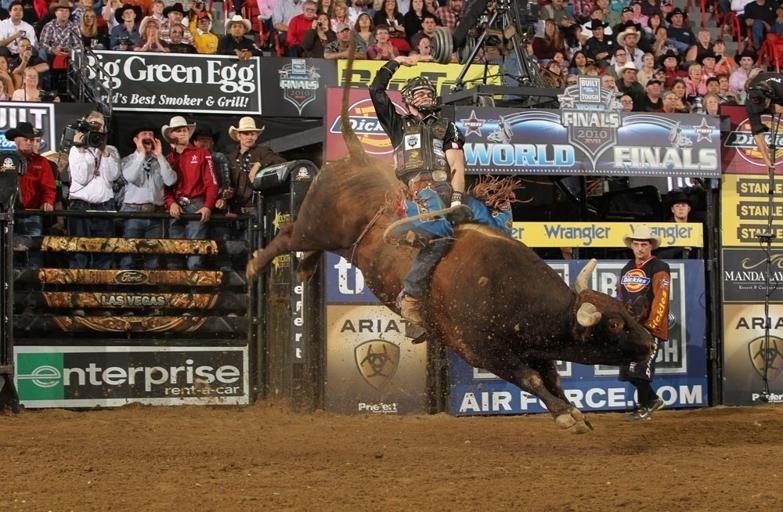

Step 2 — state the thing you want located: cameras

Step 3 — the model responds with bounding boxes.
[316,21,323,29]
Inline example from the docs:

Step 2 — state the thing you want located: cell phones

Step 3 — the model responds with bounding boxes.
[25,46,31,51]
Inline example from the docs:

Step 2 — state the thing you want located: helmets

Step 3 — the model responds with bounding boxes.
[401,77,436,112]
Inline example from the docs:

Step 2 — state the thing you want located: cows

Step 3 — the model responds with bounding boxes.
[245,28,655,436]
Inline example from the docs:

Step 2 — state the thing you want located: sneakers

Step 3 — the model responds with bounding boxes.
[648,398,665,411]
[399,296,423,322]
[626,410,651,422]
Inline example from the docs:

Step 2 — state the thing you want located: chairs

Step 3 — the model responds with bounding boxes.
[686,0,782,73]
[389,38,412,51]
[209,0,303,57]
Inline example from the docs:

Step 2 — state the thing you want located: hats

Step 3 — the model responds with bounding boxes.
[624,225,659,249]
[666,193,695,216]
[585,8,758,86]
[337,23,350,32]
[48,0,252,36]
[5,111,265,142]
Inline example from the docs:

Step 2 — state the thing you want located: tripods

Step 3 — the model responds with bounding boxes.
[448,0,538,94]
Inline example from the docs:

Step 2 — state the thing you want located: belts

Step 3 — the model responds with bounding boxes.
[128,204,155,212]
[176,197,202,206]
[421,170,448,182]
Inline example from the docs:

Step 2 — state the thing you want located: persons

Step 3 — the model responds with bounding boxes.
[366,56,497,326]
[0,0,782,168]
[613,225,671,421]
[666,192,698,259]
[4,105,287,272]
